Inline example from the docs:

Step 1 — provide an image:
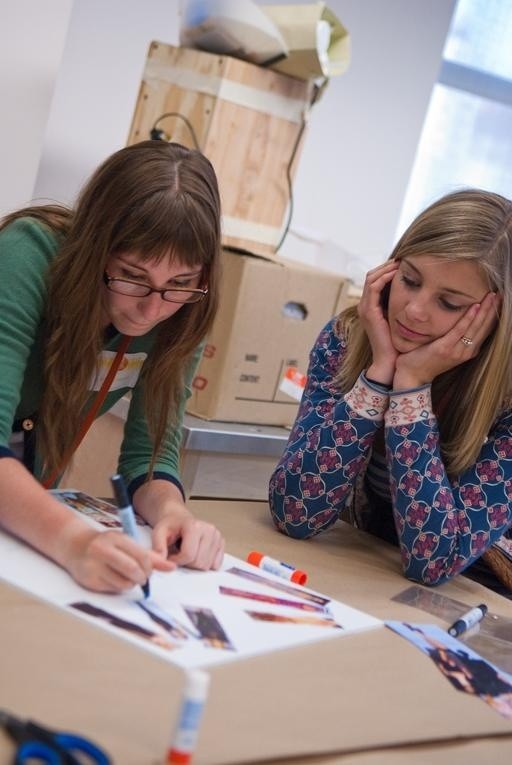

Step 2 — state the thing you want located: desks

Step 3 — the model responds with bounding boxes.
[0,498,511,762]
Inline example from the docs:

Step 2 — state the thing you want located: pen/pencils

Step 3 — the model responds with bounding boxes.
[448,604,488,636]
[110,472,151,599]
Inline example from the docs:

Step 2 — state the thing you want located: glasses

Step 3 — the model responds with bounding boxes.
[103,271,209,304]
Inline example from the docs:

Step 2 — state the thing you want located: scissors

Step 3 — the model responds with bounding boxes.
[0,710,111,765]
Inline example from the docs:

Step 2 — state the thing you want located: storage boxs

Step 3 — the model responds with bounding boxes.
[126,38,318,257]
[183,247,364,428]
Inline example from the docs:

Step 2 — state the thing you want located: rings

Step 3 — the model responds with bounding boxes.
[462,337,474,347]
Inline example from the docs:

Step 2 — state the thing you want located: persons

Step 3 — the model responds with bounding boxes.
[404,620,512,696]
[270,190,512,586]
[0,140,226,595]
